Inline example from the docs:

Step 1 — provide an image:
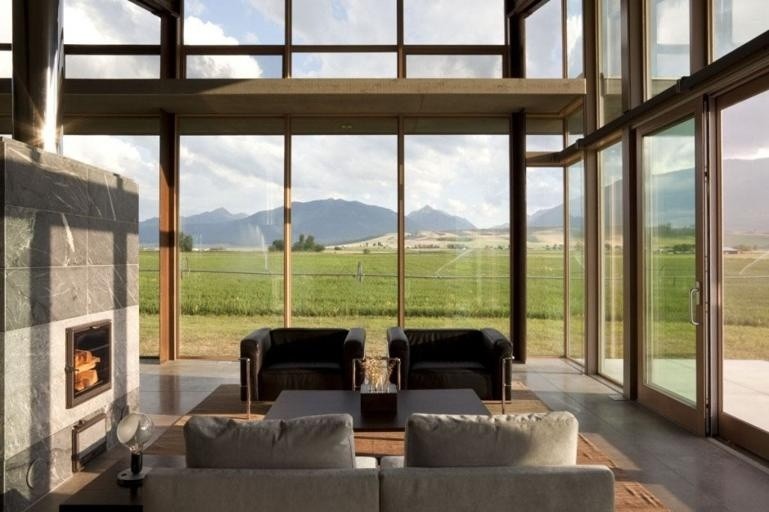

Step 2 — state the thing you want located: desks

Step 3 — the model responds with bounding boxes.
[264,389,492,459]
[58,453,187,512]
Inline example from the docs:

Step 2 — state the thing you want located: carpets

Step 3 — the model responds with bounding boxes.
[135,379,673,512]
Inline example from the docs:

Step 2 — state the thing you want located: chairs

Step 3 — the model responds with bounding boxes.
[388,326,517,400]
[242,326,366,411]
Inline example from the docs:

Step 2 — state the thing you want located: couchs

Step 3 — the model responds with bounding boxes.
[381,409,614,510]
[140,412,380,510]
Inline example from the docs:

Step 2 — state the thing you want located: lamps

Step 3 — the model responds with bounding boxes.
[116,412,154,486]
